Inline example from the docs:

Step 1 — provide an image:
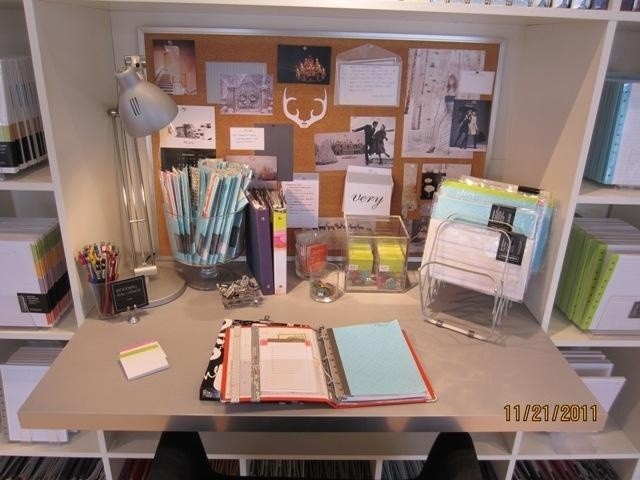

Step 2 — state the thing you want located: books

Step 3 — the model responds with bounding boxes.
[555,76,639,412]
[219,317,437,410]
[417,176,552,302]
[117,341,171,382]
[263,183,290,298]
[1,54,76,446]
[198,317,310,406]
[246,187,274,296]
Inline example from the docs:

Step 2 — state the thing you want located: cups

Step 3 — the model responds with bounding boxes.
[88,273,122,319]
[309,261,341,304]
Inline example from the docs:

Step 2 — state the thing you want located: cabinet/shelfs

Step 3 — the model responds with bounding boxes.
[0,0,640,480]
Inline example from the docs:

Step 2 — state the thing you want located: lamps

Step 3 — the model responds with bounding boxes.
[108,55,186,307]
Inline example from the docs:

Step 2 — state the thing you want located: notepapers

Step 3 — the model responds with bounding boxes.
[119,341,169,381]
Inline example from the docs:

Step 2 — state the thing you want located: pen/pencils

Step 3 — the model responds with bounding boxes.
[75,241,120,316]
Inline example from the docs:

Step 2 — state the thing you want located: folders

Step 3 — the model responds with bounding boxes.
[245,189,288,296]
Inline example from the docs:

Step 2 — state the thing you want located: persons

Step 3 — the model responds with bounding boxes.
[459,110,479,149]
[352,121,378,165]
[451,107,473,149]
[373,124,390,165]
[445,74,457,97]
